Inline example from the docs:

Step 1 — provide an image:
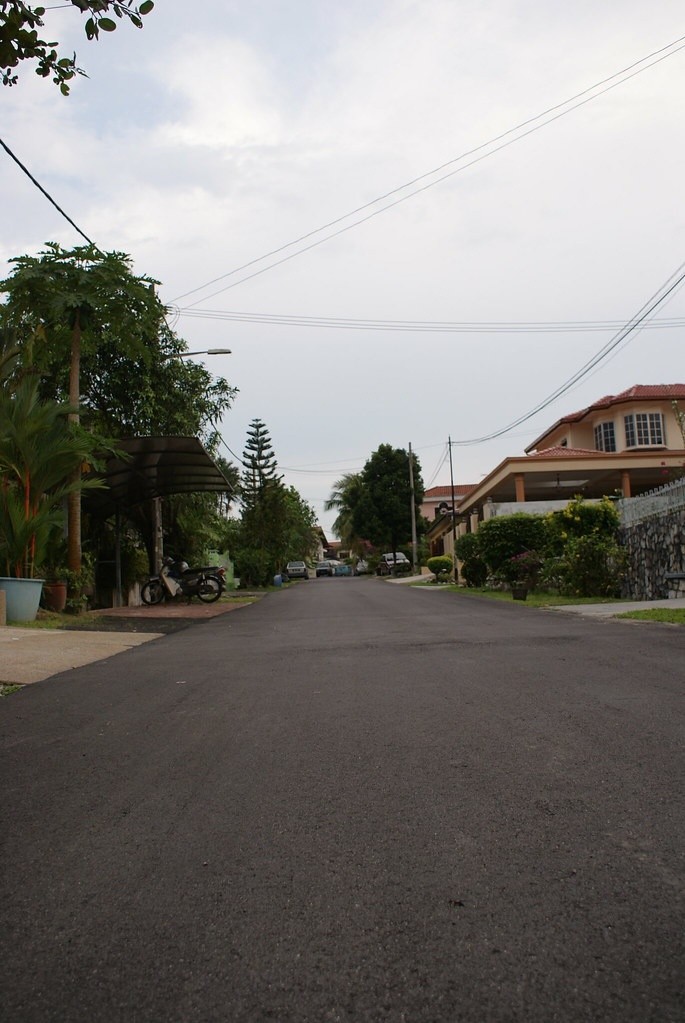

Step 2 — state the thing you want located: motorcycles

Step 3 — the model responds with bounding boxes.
[141,557,227,605]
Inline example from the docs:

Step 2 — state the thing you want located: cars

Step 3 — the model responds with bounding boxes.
[380,551,411,576]
[315,561,332,577]
[286,560,309,580]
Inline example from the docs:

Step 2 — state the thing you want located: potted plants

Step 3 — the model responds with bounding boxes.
[511,581,529,601]
[460,557,487,587]
[0,388,133,625]
[404,566,409,577]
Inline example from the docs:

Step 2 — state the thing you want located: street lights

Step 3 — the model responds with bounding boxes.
[112,348,232,607]
